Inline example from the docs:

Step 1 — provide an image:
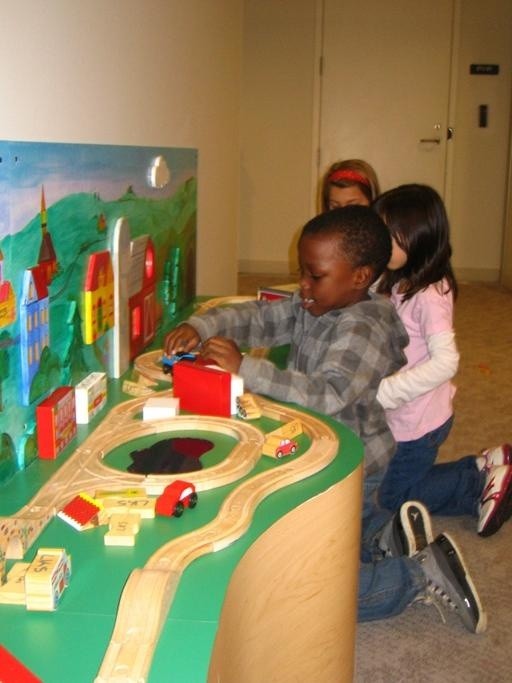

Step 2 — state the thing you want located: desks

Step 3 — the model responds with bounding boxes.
[2,292,364,682]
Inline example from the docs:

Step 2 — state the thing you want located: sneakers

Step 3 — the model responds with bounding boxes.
[477,444,512,537]
[392,501,435,558]
[413,532,486,634]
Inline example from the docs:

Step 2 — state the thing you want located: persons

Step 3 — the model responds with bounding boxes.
[159,203,490,640]
[361,181,510,545]
[321,158,381,214]
